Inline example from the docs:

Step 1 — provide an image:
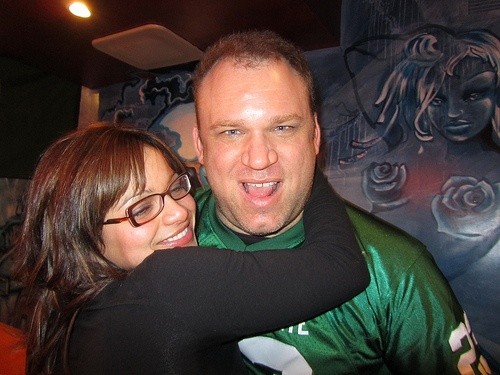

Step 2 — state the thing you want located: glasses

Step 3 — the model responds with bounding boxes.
[101,171,192,229]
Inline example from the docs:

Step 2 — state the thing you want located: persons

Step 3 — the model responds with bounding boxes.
[193,31,492,375]
[9,124,371,375]
